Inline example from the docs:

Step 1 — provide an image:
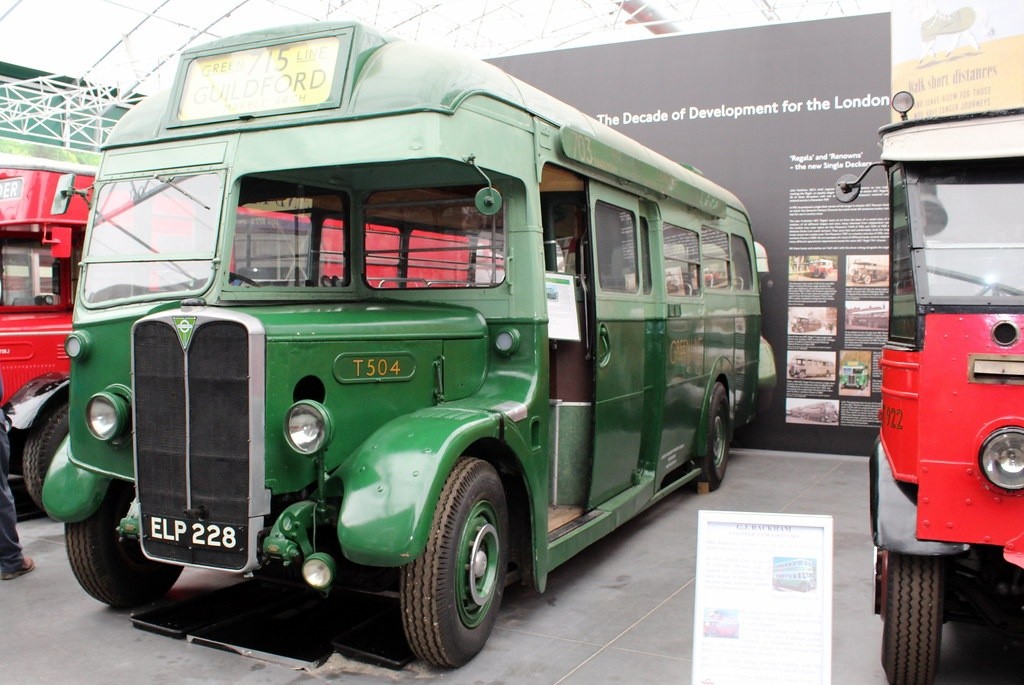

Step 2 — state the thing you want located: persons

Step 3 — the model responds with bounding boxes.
[0,374,36,581]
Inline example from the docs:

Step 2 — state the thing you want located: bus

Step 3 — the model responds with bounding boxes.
[830,86,1022,685]
[41,18,762,673]
[2,155,496,518]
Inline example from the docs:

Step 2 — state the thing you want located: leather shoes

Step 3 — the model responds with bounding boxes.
[0,556,35,580]
